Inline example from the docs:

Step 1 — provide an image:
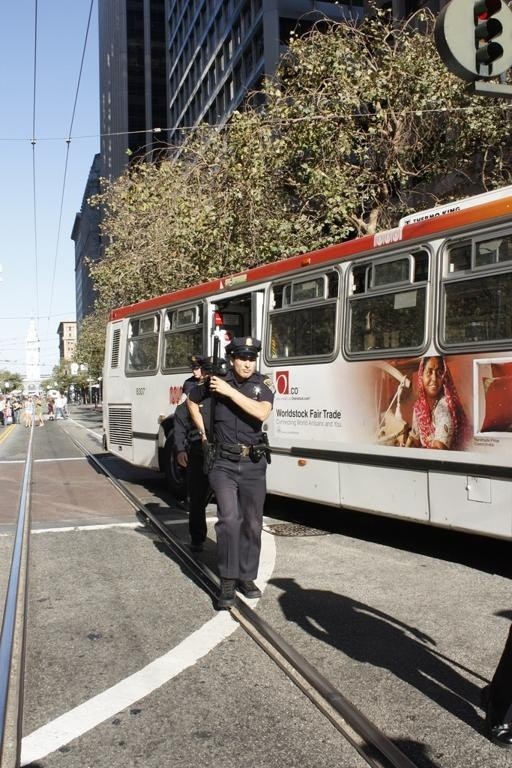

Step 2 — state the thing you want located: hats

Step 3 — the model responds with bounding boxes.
[225,337,262,359]
[200,356,226,373]
[188,355,202,369]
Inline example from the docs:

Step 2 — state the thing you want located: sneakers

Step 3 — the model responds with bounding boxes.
[236,580,261,599]
[219,583,235,607]
[189,538,204,552]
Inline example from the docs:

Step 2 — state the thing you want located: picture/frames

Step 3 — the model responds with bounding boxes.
[473,357,512,438]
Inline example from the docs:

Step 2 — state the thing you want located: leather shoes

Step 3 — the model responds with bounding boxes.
[483,684,512,748]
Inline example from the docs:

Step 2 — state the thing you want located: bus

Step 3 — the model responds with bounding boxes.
[101,183,512,542]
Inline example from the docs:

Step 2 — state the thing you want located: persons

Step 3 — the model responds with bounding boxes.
[173,357,226,552]
[187,336,274,610]
[0,389,99,427]
[405,357,472,449]
[182,355,212,401]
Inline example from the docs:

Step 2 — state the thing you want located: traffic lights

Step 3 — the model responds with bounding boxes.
[473,0,502,65]
